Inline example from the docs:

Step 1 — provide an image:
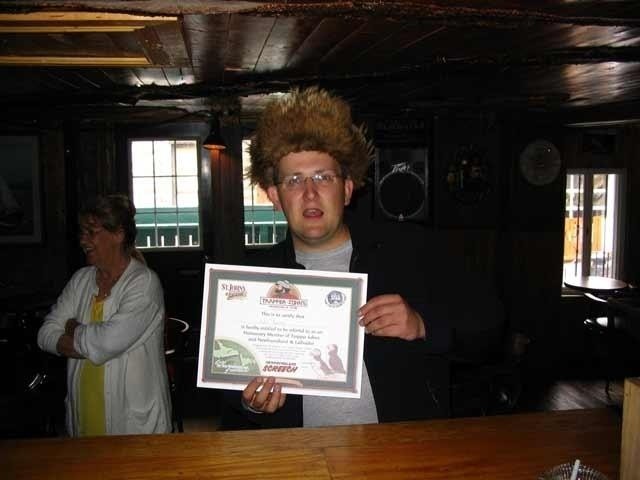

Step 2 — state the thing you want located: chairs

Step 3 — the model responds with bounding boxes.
[579,286,640,392]
[164,317,190,344]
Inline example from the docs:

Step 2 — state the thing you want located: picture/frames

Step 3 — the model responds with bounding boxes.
[1,131,46,245]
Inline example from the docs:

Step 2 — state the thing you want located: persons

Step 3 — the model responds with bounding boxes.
[222,87,505,426]
[35,194,177,435]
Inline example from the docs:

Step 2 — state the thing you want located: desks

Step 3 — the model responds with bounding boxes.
[161,344,184,432]
[564,276,628,317]
[0,405,640,480]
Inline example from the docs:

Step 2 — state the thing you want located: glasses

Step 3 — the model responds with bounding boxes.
[79,226,113,240]
[273,172,345,186]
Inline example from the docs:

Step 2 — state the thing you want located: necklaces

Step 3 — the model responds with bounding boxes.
[96,269,121,297]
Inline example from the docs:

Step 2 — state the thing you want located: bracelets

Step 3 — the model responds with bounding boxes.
[66,321,77,335]
